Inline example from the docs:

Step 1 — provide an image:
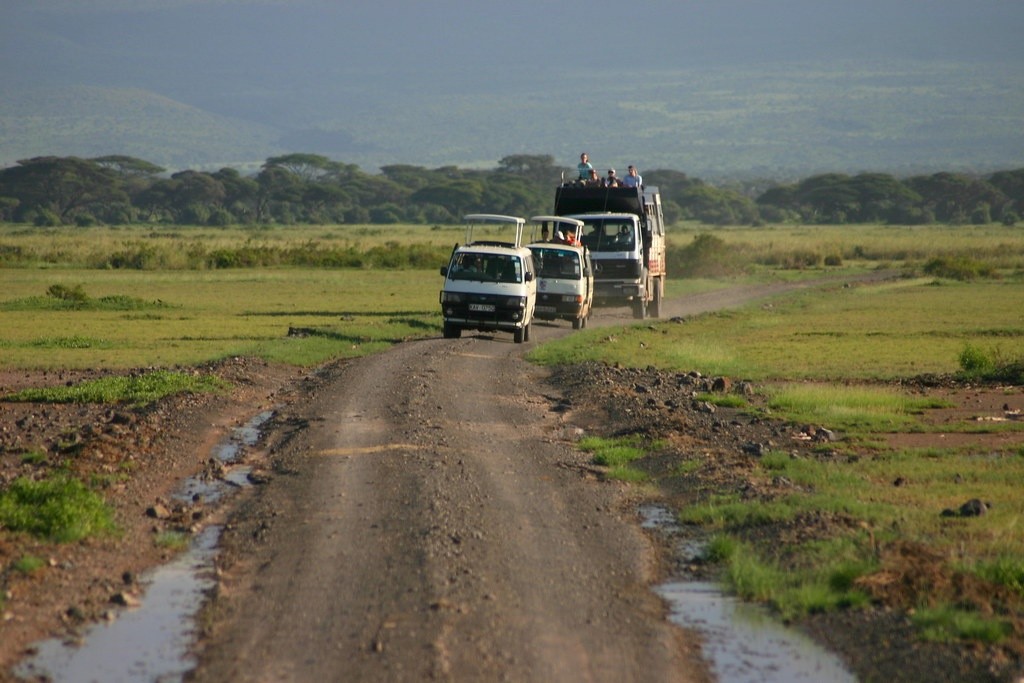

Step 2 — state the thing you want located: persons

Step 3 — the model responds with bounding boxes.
[587,226,633,244]
[569,153,643,188]
[541,228,581,247]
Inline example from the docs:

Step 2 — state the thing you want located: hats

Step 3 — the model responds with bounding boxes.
[555,231,565,241]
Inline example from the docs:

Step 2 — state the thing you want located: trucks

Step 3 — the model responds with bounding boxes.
[551,181,667,320]
[438,214,538,344]
[524,215,594,330]
